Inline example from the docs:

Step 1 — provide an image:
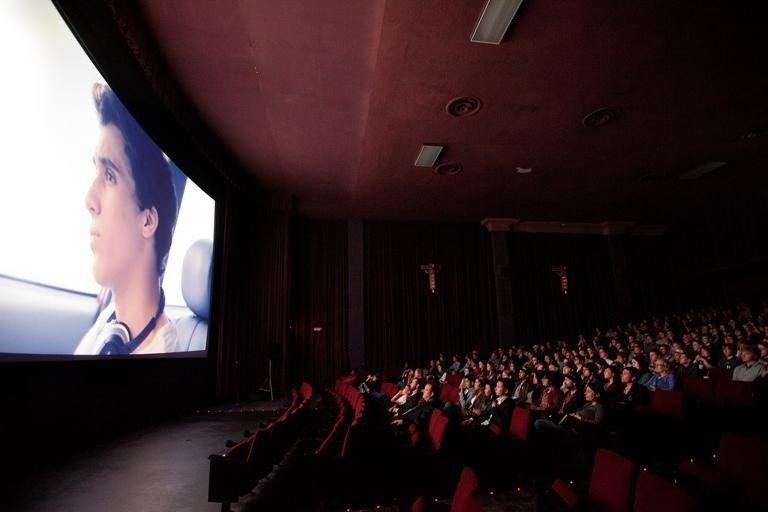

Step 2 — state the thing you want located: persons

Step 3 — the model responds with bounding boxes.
[68,82,184,356]
[342,299,767,455]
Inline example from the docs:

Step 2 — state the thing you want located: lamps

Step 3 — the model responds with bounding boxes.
[552,265,569,295]
[421,263,442,295]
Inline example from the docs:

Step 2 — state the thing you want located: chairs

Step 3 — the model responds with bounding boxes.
[168,238,214,352]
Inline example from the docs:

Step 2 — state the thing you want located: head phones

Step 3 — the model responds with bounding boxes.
[93,286,166,356]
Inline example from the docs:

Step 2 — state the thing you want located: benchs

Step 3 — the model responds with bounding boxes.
[208,364,768,512]
